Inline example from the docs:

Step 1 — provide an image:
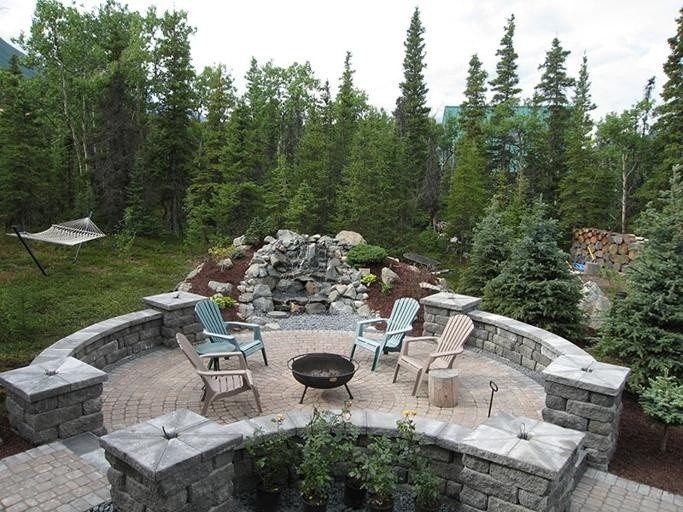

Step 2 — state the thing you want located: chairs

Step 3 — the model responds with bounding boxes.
[174,298,268,418]
[348,297,474,396]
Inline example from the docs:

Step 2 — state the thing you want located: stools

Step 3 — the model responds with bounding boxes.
[427,368,459,408]
